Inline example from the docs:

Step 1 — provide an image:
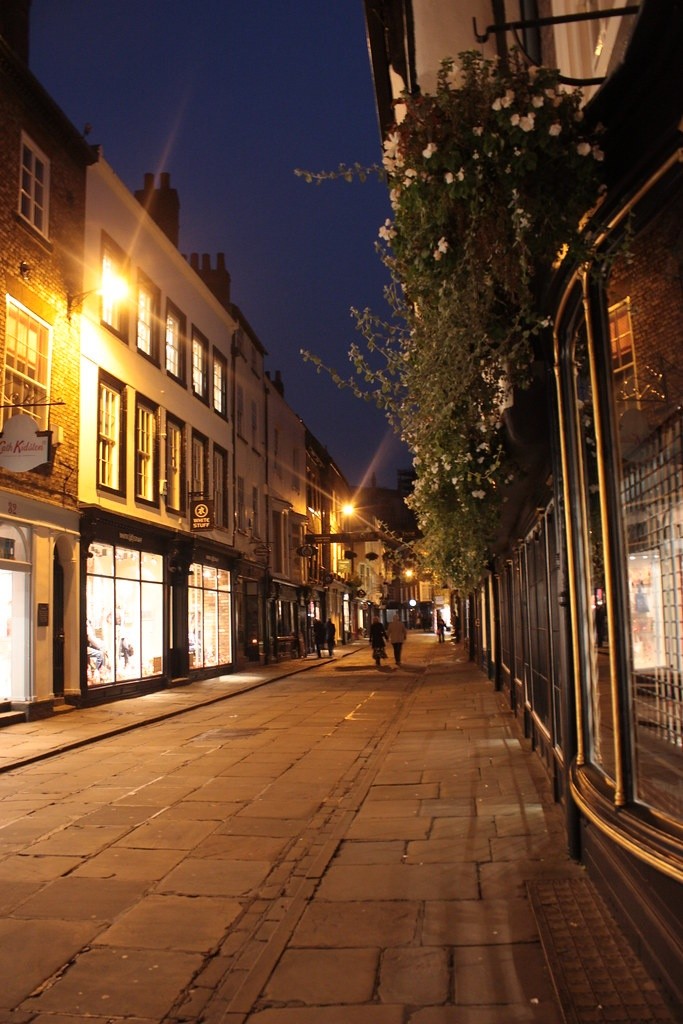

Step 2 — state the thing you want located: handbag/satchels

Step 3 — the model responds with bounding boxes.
[437,629,441,636]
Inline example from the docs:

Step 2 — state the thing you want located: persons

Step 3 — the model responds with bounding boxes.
[325,617,335,656]
[387,615,406,665]
[595,604,604,648]
[369,616,388,666]
[86,612,134,681]
[189,610,201,662]
[422,614,432,631]
[437,616,448,642]
[451,611,460,644]
[409,614,415,629]
[312,618,326,657]
